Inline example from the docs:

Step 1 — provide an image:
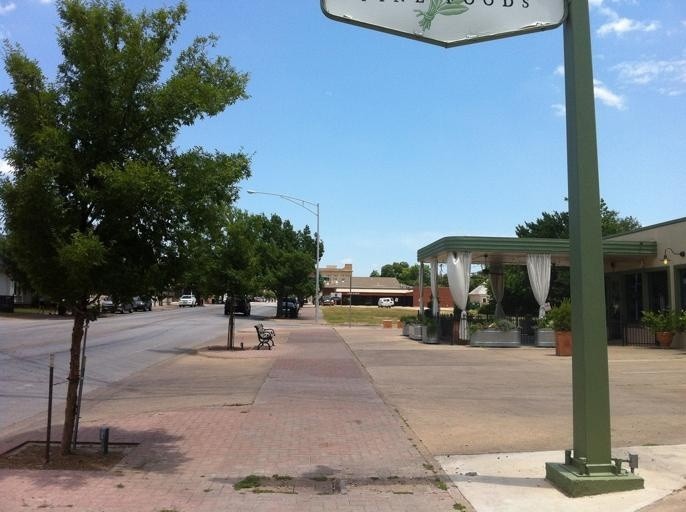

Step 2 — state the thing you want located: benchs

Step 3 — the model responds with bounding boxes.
[253,323,275,352]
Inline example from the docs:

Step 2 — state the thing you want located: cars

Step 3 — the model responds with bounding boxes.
[131,296,152,312]
[102,296,135,314]
[332,297,350,305]
[323,296,335,306]
[254,296,266,302]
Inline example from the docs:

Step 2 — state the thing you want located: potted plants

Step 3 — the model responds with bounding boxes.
[540,298,573,357]
[530,318,557,349]
[638,304,685,347]
[470,319,523,347]
[421,318,440,344]
[409,317,422,340]
[399,315,412,337]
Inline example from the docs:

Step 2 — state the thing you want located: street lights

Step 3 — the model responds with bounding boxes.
[246,189,320,323]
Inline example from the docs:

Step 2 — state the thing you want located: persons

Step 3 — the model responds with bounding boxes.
[394,296,398,305]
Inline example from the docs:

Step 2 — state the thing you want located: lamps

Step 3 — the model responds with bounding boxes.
[660,246,685,266]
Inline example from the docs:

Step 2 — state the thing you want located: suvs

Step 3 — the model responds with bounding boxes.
[274,297,299,319]
[377,297,394,308]
[178,294,197,307]
[224,294,252,316]
